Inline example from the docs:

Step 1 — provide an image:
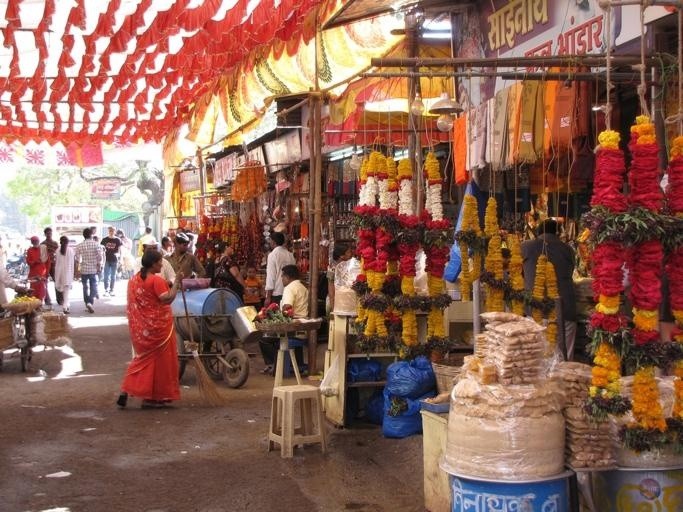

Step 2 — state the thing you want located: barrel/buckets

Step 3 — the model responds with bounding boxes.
[168,286,242,337]
[588,469,683,511]
[230,305,262,343]
[448,474,572,511]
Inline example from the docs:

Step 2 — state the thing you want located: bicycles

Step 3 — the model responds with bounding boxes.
[9,274,45,370]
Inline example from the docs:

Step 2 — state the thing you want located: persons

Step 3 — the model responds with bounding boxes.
[117,249,184,409]
[259,263,309,374]
[520,217,575,361]
[242,265,263,313]
[327,243,352,324]
[264,231,296,310]
[26,236,50,303]
[40,227,64,306]
[53,226,200,314]
[0,238,27,309]
[213,241,249,305]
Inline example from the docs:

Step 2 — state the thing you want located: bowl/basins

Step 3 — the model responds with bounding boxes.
[182,278,212,289]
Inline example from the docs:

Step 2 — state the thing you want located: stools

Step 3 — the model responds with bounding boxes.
[268,385,326,459]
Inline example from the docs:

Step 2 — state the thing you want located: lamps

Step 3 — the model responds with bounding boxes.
[350,132,361,169]
[428,75,464,132]
[411,77,425,115]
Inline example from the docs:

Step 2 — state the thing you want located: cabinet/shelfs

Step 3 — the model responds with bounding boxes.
[326,310,426,427]
[334,196,358,243]
[443,300,474,365]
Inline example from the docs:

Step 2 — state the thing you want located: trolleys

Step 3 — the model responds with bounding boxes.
[172,312,249,390]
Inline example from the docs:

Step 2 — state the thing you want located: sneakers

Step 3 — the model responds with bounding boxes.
[259,365,272,373]
[62,308,71,315]
[141,398,163,409]
[116,395,127,406]
[87,303,94,313]
[103,290,115,297]
